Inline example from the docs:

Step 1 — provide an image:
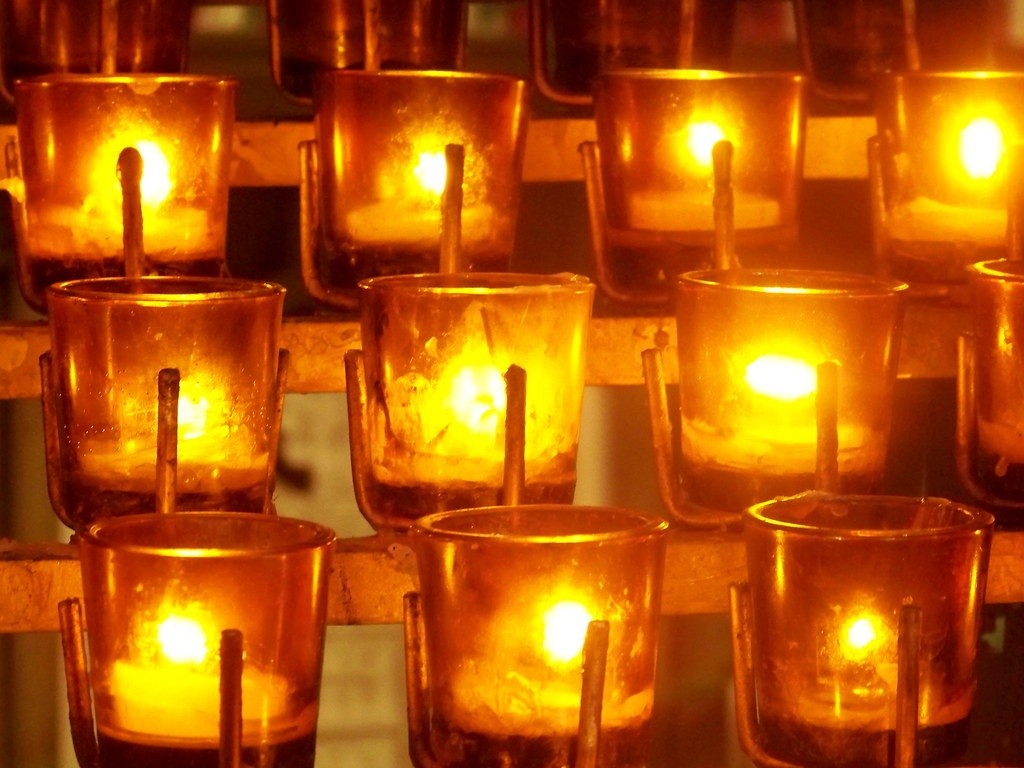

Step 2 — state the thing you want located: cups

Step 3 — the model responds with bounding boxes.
[0,2,1024,767]
